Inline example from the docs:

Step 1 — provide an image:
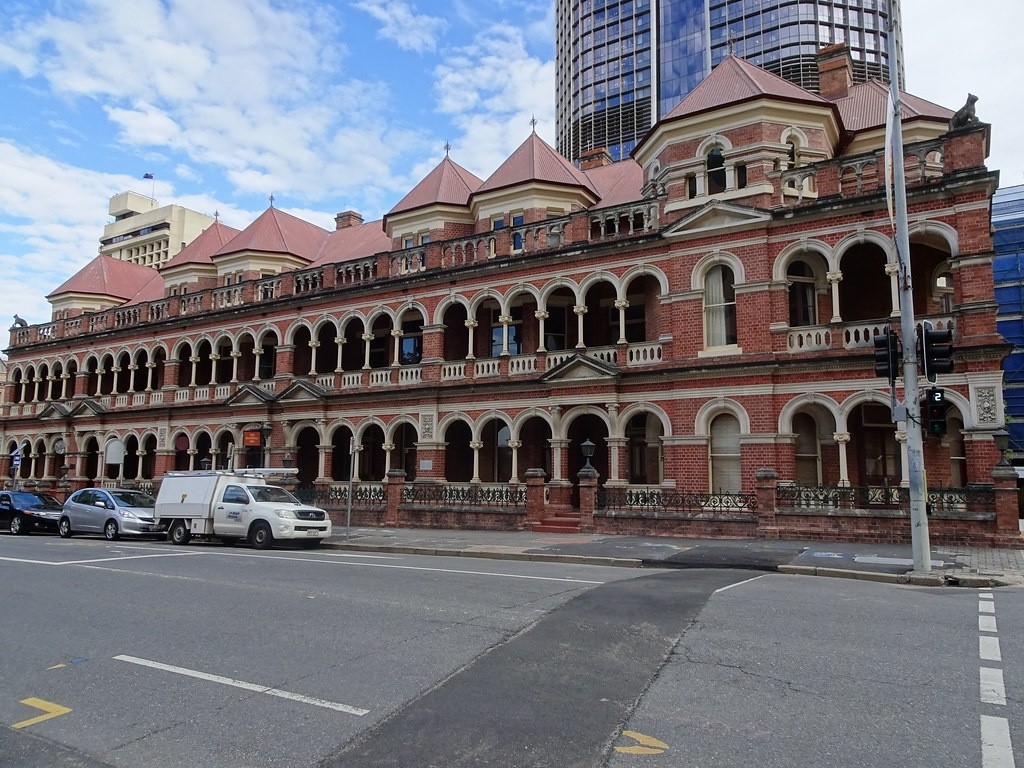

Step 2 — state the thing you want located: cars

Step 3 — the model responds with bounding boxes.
[57,487,170,541]
[0,490,64,535]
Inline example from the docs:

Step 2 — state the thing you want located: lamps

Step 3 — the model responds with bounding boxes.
[581,438,595,470]
[60,464,69,482]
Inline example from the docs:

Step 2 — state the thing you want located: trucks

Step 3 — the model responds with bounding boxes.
[154,466,334,551]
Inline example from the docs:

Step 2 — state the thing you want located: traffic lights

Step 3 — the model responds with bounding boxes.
[926,387,947,437]
[922,320,955,382]
[873,326,899,386]
